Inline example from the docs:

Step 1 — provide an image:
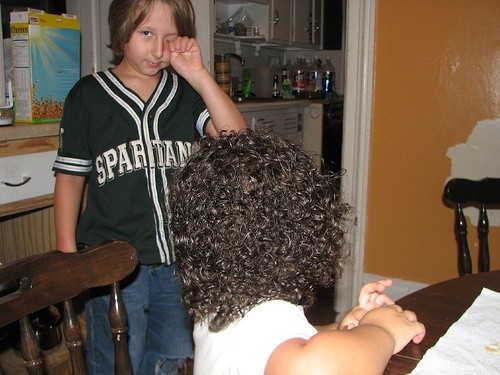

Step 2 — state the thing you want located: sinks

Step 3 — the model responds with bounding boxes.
[231,96,273,102]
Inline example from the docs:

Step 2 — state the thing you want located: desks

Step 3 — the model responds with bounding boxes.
[382,270,500,375]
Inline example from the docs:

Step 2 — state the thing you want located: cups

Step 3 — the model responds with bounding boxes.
[214,54,230,96]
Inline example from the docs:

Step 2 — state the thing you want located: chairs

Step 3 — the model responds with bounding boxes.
[0,241,139,375]
[444,177,500,277]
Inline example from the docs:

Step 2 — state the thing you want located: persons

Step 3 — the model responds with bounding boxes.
[51,0,250,374]
[171,128,425,375]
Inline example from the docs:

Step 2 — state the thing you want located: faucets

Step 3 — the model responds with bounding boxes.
[224,52,245,96]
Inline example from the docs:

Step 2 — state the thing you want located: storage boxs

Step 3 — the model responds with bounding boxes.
[10,8,80,123]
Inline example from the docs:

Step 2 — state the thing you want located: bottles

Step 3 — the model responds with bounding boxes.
[272,75,280,99]
[229,18,235,35]
[245,26,251,36]
[281,67,291,100]
[285,58,294,99]
[311,56,322,100]
[254,27,259,37]
[292,56,316,100]
[322,56,335,99]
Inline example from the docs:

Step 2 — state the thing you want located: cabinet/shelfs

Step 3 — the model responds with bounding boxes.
[0,141,60,209]
[239,106,304,145]
[190,0,324,53]
